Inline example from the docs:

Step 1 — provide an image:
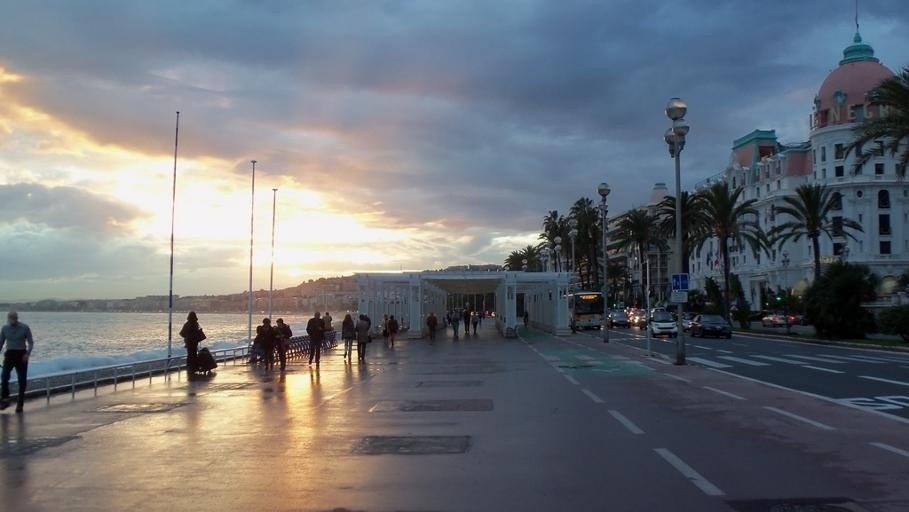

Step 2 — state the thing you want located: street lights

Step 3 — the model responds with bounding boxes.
[595,181,613,345]
[568,217,581,330]
[521,258,529,272]
[539,247,550,272]
[663,94,691,364]
[554,235,563,271]
[781,250,791,289]
[838,245,850,263]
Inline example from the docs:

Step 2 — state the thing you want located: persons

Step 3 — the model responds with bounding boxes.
[179,311,199,374]
[247,305,483,371]
[523,308,529,325]
[0,311,34,414]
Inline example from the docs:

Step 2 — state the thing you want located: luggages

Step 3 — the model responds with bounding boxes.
[197,347,217,372]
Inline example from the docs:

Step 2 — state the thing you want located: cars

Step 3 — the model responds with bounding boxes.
[681,312,701,332]
[688,314,732,340]
[762,312,795,328]
[605,305,679,327]
[792,313,811,326]
[650,310,677,339]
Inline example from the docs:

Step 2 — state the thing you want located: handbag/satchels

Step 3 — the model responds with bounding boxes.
[198,328,206,341]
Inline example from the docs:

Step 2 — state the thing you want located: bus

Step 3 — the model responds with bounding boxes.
[563,291,604,331]
